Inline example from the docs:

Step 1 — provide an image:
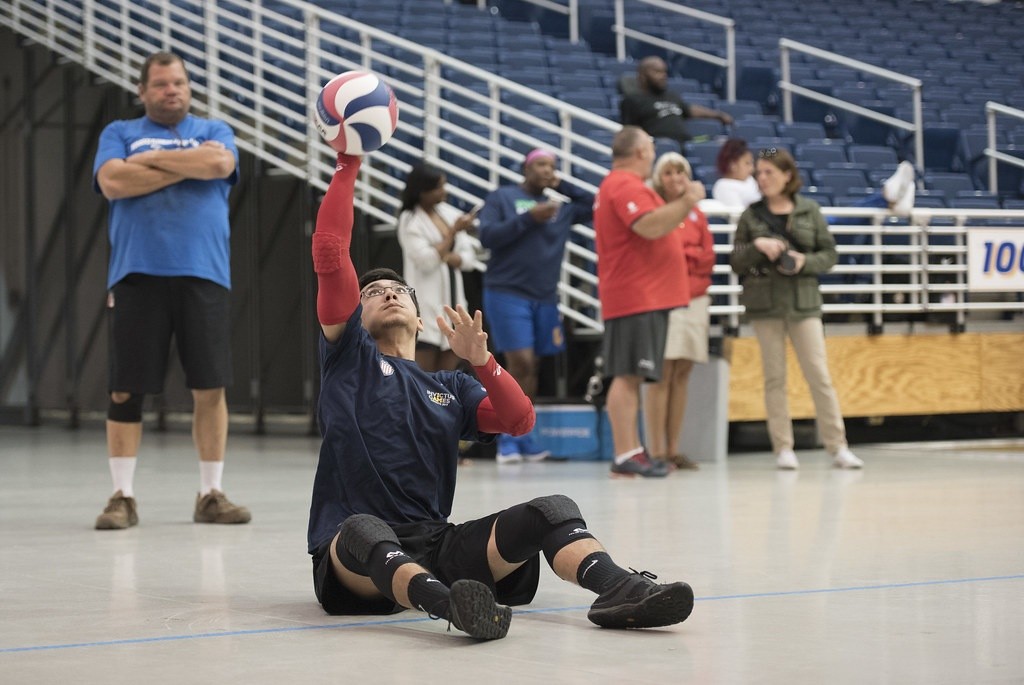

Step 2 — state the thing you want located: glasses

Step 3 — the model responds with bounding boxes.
[359,285,417,304]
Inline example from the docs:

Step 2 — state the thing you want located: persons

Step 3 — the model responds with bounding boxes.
[594,126,706,479]
[712,136,916,243]
[397,162,476,374]
[307,153,694,642]
[476,144,595,464]
[730,147,864,470]
[619,55,733,153]
[94,52,252,530]
[648,152,716,471]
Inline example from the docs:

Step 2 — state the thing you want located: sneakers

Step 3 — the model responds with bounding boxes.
[587,566,695,628]
[496,431,523,465]
[515,436,550,463]
[607,448,676,481]
[192,490,252,524]
[666,454,701,473]
[429,579,512,640]
[95,490,138,529]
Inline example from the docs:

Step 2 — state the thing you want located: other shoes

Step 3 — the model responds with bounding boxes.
[776,445,797,469]
[833,445,867,470]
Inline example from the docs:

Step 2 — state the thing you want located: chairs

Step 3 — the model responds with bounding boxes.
[37,0,1024,311]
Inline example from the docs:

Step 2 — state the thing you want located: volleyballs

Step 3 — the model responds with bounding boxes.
[311,69,401,154]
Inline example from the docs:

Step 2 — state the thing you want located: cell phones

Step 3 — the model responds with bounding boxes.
[469,202,485,216]
